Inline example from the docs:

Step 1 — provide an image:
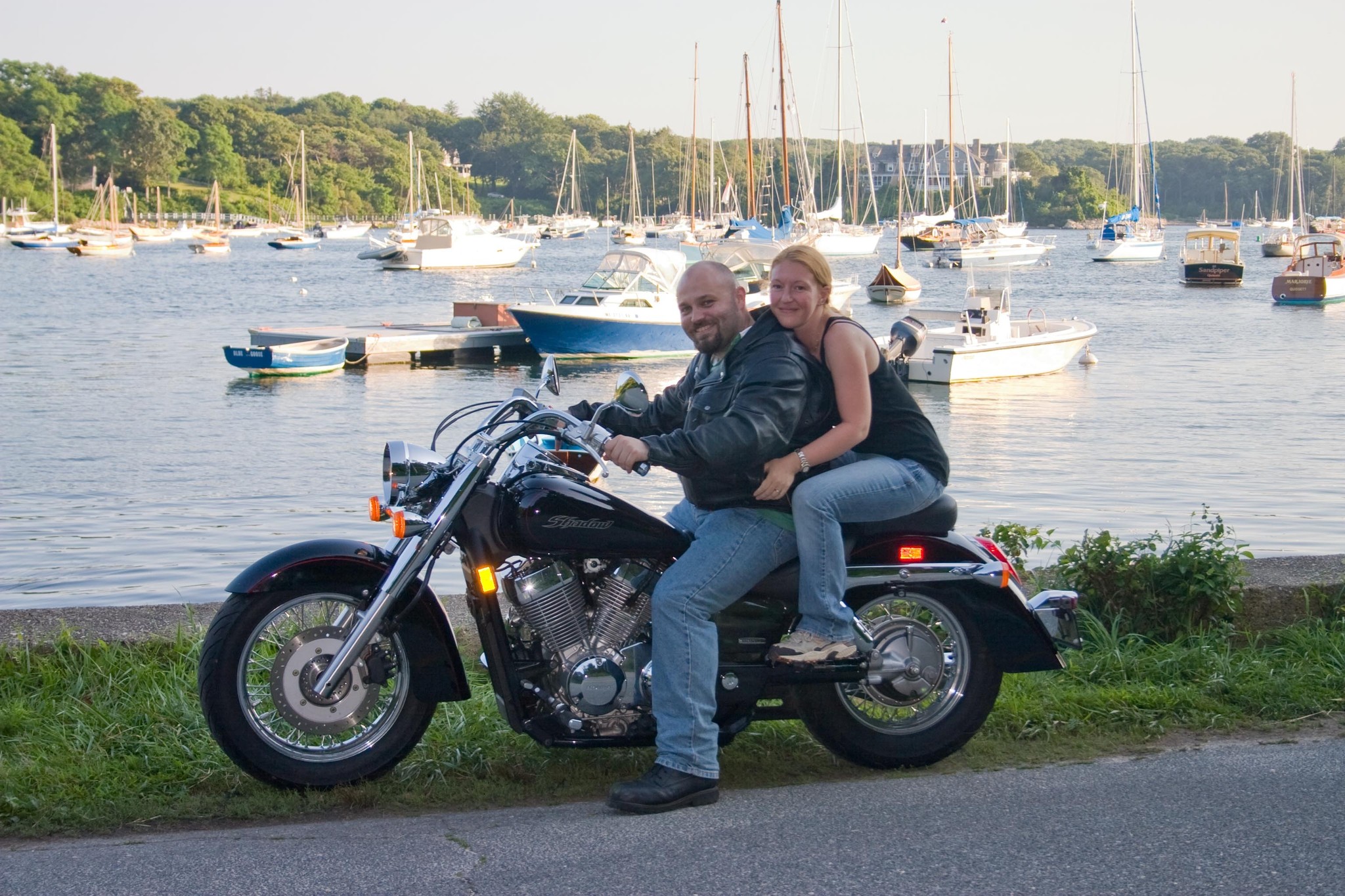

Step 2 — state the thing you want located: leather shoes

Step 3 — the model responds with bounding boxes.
[607,762,720,812]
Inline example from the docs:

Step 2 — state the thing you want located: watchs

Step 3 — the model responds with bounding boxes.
[794,448,811,473]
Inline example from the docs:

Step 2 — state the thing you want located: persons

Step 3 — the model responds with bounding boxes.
[754,245,949,665]
[528,261,842,814]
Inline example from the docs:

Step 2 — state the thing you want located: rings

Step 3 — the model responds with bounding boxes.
[774,489,780,494]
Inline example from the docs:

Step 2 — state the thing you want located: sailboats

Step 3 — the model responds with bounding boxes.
[1180,72,1345,308]
[0,1,1058,271]
[1086,1,1168,261]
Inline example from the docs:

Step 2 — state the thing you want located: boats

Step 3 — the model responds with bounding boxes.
[504,247,861,359]
[221,336,349,377]
[878,286,1098,383]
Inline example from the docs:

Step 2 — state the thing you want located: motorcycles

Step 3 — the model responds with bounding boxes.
[198,353,1081,789]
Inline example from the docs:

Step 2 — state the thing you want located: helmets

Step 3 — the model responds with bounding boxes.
[533,439,599,476]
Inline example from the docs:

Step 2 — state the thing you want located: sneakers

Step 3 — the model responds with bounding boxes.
[767,628,859,664]
